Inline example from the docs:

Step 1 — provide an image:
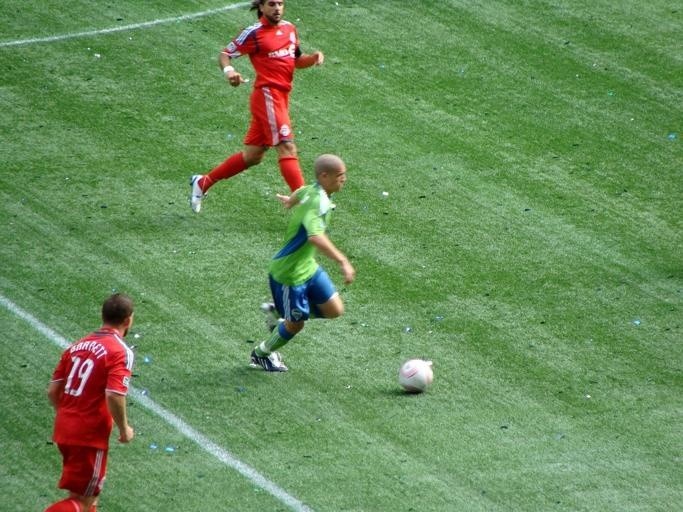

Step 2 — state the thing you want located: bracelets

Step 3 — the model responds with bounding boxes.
[223,65,235,73]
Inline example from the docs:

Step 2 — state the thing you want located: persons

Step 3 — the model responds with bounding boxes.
[187,1,327,216]
[250,153,357,373]
[40,290,137,512]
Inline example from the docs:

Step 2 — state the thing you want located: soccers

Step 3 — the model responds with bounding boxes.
[399,359,434,392]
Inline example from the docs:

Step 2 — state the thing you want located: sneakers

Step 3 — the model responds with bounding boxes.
[189,175,208,214]
[262,302,280,320]
[251,346,288,372]
